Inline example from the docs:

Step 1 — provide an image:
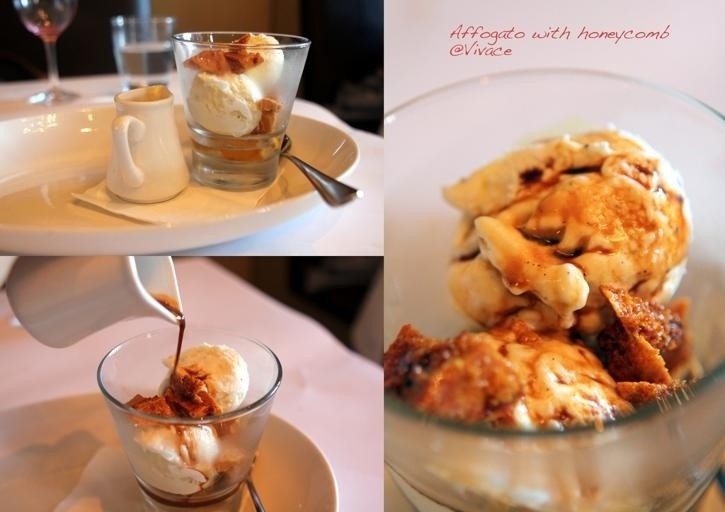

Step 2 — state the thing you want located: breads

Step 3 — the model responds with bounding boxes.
[184,33,285,137]
[441,130,689,331]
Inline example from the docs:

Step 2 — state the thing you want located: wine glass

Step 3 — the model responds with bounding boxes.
[14,0,79,104]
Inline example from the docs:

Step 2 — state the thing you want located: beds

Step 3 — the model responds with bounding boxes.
[1,71,384,256]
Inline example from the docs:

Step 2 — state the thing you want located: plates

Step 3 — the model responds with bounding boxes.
[0,101,358,256]
[0,389,339,512]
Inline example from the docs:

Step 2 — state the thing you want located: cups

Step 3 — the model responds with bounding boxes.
[108,84,190,203]
[95,326,283,512]
[5,256,184,349]
[384,67,724,512]
[170,31,311,191]
[111,17,174,88]
